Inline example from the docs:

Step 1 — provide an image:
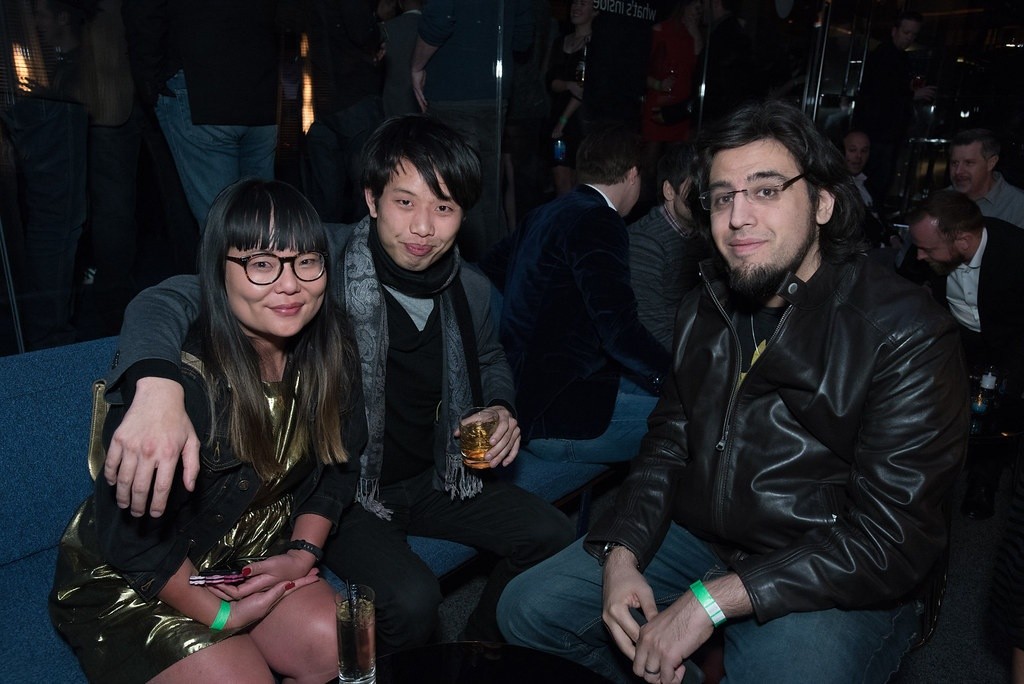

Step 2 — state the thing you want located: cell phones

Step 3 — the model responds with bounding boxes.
[189,571,245,585]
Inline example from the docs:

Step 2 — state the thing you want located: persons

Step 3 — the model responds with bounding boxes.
[45,180,371,684]
[945,127,1024,230]
[825,118,904,248]
[496,123,672,465]
[848,7,942,191]
[0,1,206,351]
[276,0,818,299]
[898,190,1024,521]
[625,148,715,355]
[494,100,972,683]
[118,1,288,234]
[104,110,623,654]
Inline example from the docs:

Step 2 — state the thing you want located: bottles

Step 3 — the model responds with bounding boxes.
[553,137,566,161]
[971,371,996,435]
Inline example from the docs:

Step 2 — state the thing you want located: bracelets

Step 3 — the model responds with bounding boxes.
[688,580,727,627]
[209,600,231,633]
[285,539,323,561]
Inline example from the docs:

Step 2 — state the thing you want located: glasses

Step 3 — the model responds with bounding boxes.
[226,249,328,286]
[697,173,805,211]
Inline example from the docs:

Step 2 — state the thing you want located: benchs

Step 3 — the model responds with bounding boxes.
[0,242,611,684]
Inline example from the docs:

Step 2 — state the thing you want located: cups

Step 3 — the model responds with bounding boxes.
[334,584,377,684]
[912,74,927,91]
[458,406,501,470]
